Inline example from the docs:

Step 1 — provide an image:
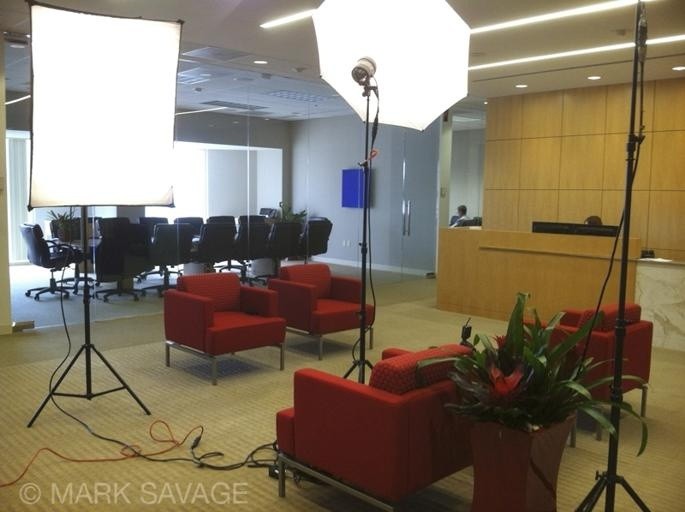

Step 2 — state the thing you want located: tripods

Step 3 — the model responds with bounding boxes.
[343,80,373,384]
[27,205,150,427]
[573,0,652,512]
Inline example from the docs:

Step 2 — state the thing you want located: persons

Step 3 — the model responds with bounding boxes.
[451,204,470,226]
[584,215,603,225]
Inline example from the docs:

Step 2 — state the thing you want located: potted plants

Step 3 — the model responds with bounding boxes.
[417,288,649,511]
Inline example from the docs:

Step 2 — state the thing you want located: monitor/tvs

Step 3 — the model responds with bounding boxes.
[533,221,571,234]
[572,224,618,236]
[342,168,370,207]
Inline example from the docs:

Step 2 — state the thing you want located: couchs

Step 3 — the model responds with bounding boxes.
[268,264,376,360]
[162,272,286,387]
[497,303,652,440]
[276,344,484,511]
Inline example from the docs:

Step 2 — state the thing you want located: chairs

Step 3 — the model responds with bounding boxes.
[20,208,332,302]
